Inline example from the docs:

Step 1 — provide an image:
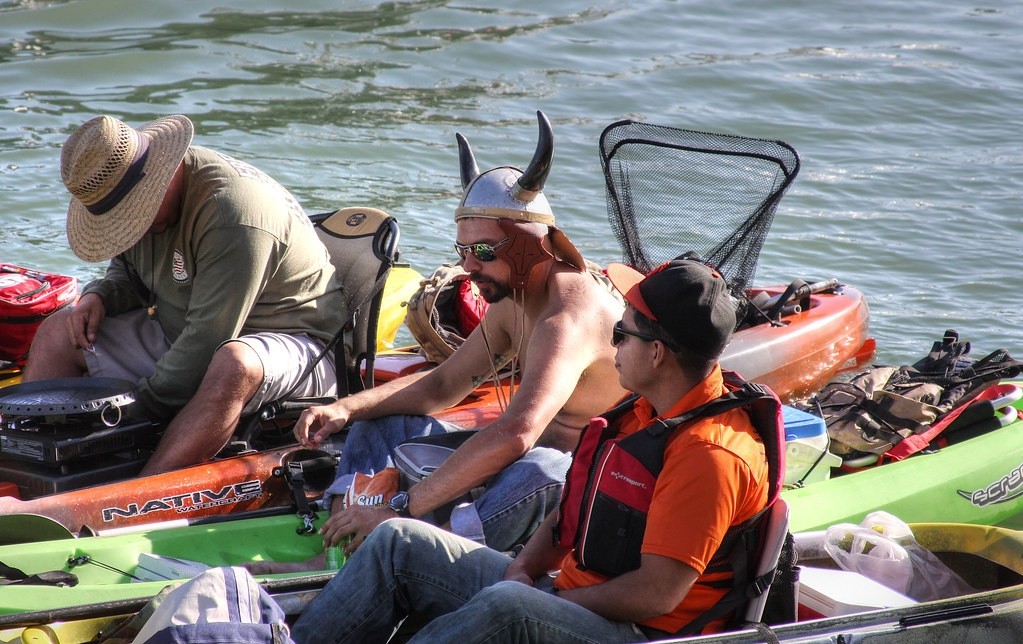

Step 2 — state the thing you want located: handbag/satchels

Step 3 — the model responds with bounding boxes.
[823,511,980,602]
[135,566,294,644]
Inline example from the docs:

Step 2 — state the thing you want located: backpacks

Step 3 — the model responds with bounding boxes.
[0,262,79,363]
[811,364,957,459]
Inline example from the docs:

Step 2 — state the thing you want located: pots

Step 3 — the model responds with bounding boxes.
[0,377,136,425]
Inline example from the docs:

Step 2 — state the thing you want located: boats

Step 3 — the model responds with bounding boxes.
[1,116,1023,644]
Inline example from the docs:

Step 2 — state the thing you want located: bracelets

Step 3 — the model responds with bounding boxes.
[543,586,560,597]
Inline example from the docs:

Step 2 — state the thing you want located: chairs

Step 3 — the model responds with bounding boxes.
[221,207,400,458]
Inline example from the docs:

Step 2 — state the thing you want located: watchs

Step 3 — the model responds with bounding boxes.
[388,491,414,518]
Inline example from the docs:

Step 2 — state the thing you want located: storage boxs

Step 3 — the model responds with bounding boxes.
[798,565,920,621]
[781,404,842,494]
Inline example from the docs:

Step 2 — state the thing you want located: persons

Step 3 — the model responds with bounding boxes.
[288,260,785,644]
[237,111,630,574]
[21,116,354,479]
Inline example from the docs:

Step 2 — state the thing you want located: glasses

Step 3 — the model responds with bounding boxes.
[454,235,509,262]
[613,321,682,357]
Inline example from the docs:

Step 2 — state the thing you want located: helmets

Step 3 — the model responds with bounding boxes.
[454,106,557,228]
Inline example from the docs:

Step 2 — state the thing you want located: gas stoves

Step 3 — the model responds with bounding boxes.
[0,415,153,476]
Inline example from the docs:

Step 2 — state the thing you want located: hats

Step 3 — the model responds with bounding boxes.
[59,113,196,262]
[605,262,737,360]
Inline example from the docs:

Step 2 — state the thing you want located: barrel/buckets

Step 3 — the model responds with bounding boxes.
[394,443,488,528]
